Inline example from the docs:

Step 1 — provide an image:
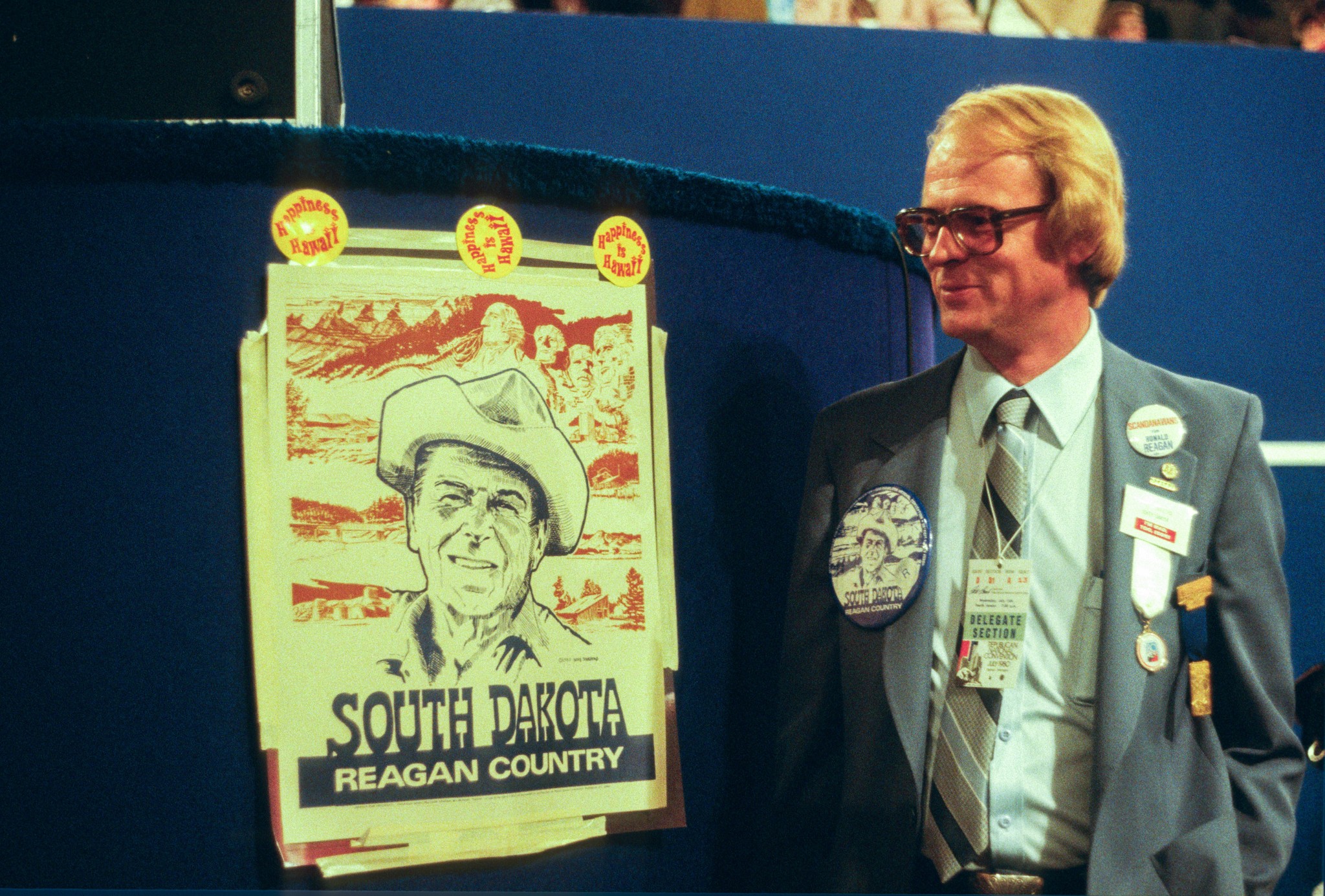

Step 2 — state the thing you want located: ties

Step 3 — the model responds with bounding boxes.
[912,399,1037,884]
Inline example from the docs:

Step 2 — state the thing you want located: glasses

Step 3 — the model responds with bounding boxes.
[896,202,1050,256]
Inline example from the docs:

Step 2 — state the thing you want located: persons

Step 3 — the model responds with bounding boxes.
[1096,3,1148,40]
[767,82,1306,895]
[1291,9,1325,55]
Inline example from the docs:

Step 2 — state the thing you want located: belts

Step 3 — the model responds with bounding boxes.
[918,867,1091,896]
